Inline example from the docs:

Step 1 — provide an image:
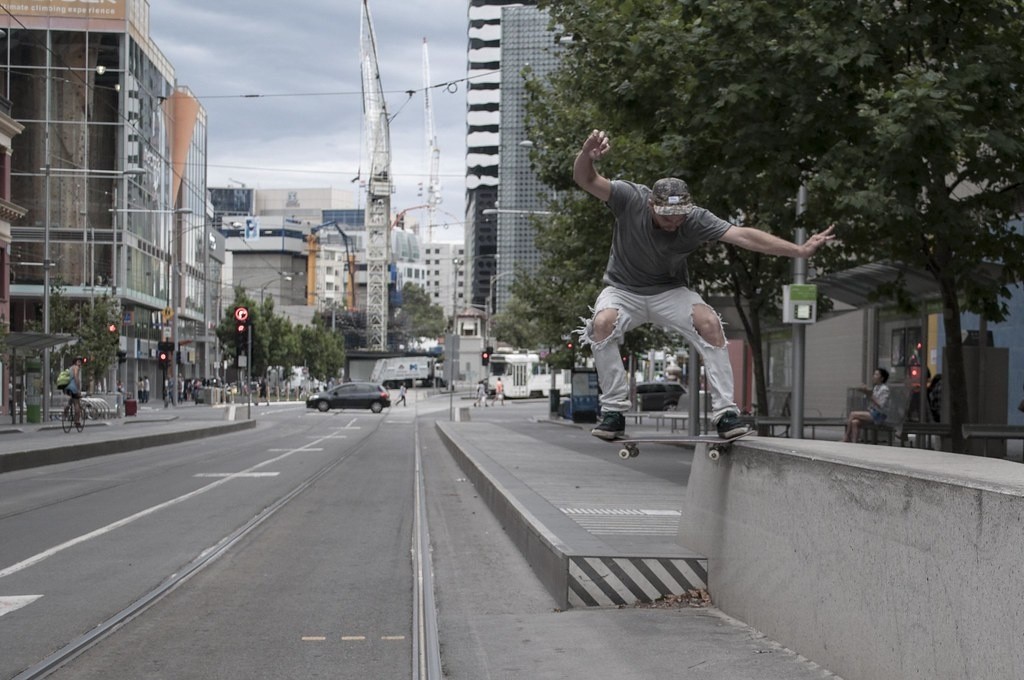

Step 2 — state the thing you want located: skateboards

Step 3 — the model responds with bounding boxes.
[592,430,755,461]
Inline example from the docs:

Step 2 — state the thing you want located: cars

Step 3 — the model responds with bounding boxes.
[306,382,391,413]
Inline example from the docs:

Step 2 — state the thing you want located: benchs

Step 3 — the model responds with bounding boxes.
[622,412,714,435]
[901,422,951,452]
[862,426,897,446]
[758,416,850,444]
[740,390,792,437]
[962,424,1024,459]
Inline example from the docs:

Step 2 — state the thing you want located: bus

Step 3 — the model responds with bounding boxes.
[487,347,572,398]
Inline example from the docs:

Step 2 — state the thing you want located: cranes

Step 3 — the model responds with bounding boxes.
[358,0,398,353]
[422,37,447,245]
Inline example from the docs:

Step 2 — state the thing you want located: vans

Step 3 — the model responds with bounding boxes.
[635,382,687,411]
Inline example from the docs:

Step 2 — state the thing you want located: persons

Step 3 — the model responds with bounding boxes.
[395,382,407,406]
[138,377,143,403]
[62,356,81,425]
[473,380,489,407]
[491,377,506,406]
[8,380,12,415]
[574,129,835,443]
[163,373,270,409]
[144,375,149,403]
[117,380,129,395]
[842,368,890,443]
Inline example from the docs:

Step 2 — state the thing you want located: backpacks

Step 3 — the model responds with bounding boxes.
[56,365,80,389]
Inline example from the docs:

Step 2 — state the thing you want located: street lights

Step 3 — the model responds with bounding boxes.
[40,168,147,422]
[108,207,192,392]
[261,275,292,316]
[172,221,242,404]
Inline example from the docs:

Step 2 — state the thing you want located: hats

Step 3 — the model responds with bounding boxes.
[651,178,693,216]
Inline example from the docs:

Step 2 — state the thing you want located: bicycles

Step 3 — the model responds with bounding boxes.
[55,397,110,421]
[62,394,92,434]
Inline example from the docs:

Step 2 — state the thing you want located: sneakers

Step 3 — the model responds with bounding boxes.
[717,411,749,438]
[592,412,626,437]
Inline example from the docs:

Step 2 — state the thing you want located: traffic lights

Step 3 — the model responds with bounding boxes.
[108,320,120,344]
[159,352,168,368]
[621,354,629,370]
[566,340,574,349]
[482,352,489,366]
[81,355,88,365]
[176,351,181,363]
[236,323,248,352]
[118,351,126,364]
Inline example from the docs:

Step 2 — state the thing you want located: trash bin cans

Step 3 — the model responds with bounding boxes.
[551,388,560,412]
[699,391,711,415]
